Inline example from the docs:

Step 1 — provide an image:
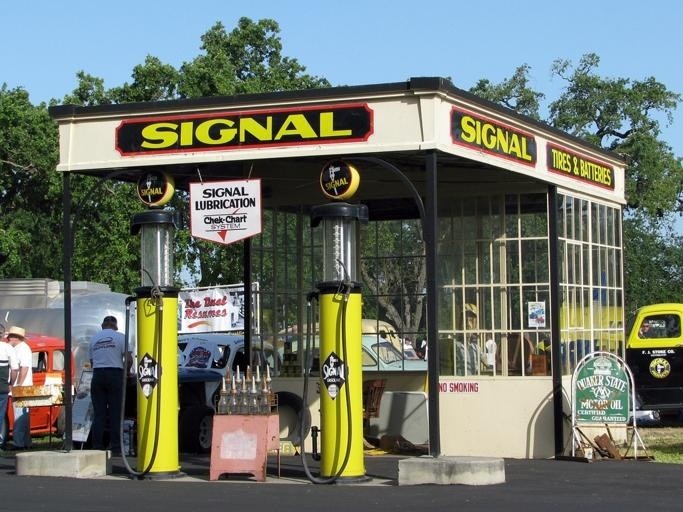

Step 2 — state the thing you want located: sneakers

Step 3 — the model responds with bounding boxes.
[4,444,24,451]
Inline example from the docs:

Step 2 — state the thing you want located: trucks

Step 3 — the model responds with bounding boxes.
[627,303,683,414]
[558,302,623,365]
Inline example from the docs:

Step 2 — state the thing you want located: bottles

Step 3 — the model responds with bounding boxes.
[218,365,272,415]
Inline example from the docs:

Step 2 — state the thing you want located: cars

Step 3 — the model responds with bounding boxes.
[178,319,428,455]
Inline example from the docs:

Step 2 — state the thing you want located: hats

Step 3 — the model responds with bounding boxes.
[5,325,26,338]
[461,303,480,316]
[101,315,118,329]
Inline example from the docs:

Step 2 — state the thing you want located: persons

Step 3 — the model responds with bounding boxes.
[638,320,659,338]
[88,316,132,457]
[0,324,19,448]
[402,337,413,350]
[6,326,33,451]
[484,332,497,367]
[451,303,482,376]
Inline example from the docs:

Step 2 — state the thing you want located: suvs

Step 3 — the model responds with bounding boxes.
[0,333,78,444]
